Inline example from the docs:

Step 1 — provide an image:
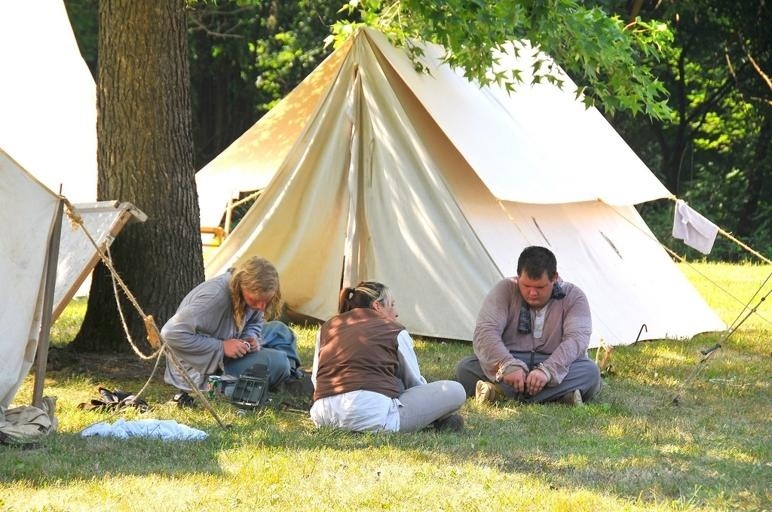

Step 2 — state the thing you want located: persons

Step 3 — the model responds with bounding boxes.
[312,279,467,436]
[158,254,302,397]
[454,244,602,408]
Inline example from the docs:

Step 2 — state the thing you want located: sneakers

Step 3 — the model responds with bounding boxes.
[475,380,498,402]
[564,389,582,406]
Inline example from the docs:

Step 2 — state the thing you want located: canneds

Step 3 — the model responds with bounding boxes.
[207,375,222,402]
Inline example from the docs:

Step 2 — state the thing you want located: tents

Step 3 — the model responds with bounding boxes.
[2,141,147,444]
[193,27,735,347]
[2,0,102,301]
